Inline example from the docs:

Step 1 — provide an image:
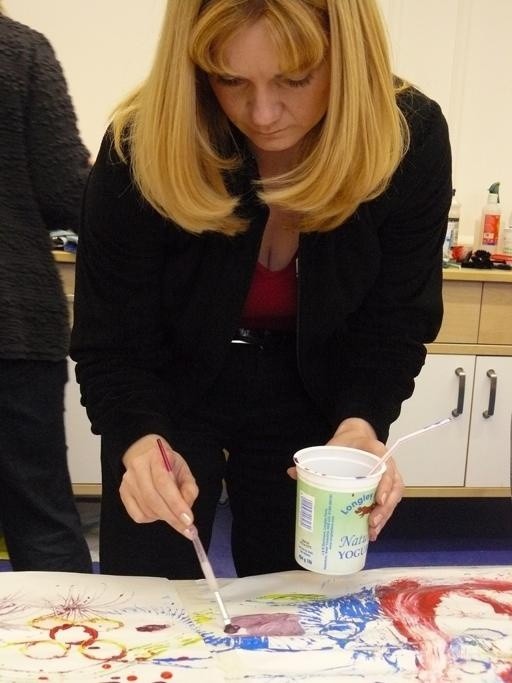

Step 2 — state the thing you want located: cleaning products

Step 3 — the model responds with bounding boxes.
[443,187,460,249]
[479,182,502,253]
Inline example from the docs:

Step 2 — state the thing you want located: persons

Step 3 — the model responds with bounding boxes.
[68,1,457,580]
[1,1,94,574]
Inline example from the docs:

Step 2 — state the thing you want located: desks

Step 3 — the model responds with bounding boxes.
[0,566,512,682]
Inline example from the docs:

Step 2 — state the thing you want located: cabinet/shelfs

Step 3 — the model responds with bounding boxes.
[56,251,512,498]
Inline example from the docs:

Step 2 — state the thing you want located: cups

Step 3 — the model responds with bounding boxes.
[288,445,387,578]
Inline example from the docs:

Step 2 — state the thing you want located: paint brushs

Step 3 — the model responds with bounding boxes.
[155,439,237,633]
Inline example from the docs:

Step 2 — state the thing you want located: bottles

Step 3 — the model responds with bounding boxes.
[441,186,462,262]
